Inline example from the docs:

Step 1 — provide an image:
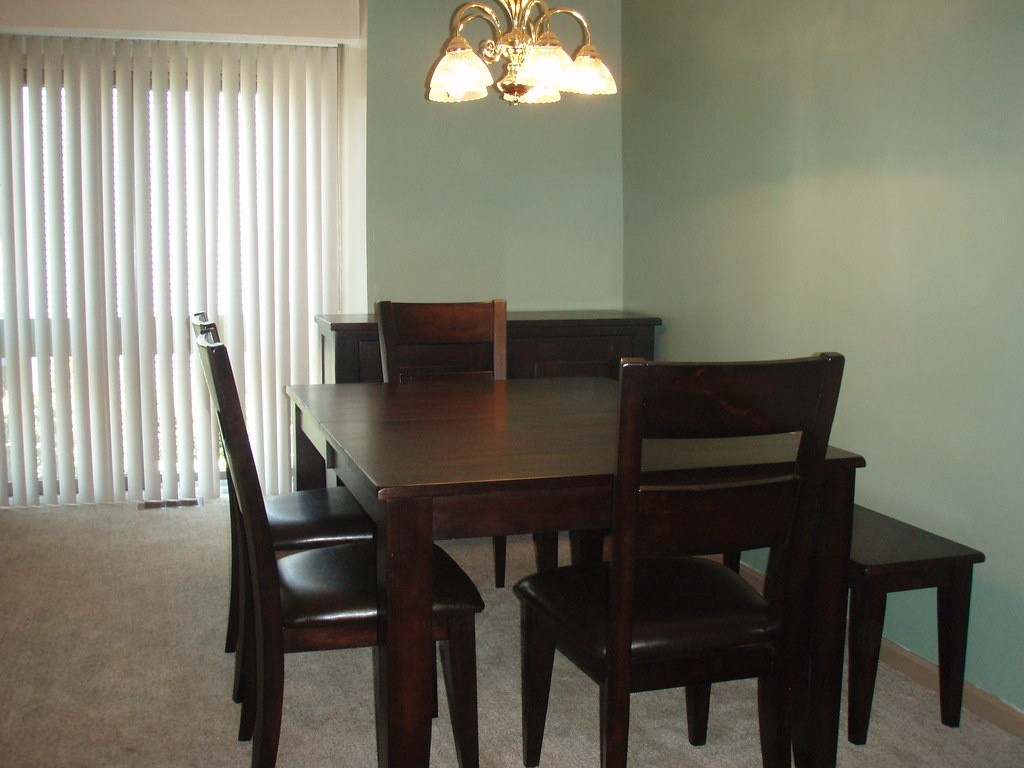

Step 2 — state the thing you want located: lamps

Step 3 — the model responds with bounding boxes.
[426,0,619,109]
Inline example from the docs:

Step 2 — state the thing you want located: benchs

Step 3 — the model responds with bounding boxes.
[723,503,987,746]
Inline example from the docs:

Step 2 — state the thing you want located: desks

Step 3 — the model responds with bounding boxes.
[283,374,867,768]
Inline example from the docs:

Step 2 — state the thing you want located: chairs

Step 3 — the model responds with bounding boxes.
[372,300,510,589]
[190,310,485,768]
[512,350,846,768]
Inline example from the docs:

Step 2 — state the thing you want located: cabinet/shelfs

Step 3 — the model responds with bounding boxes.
[312,307,664,383]
